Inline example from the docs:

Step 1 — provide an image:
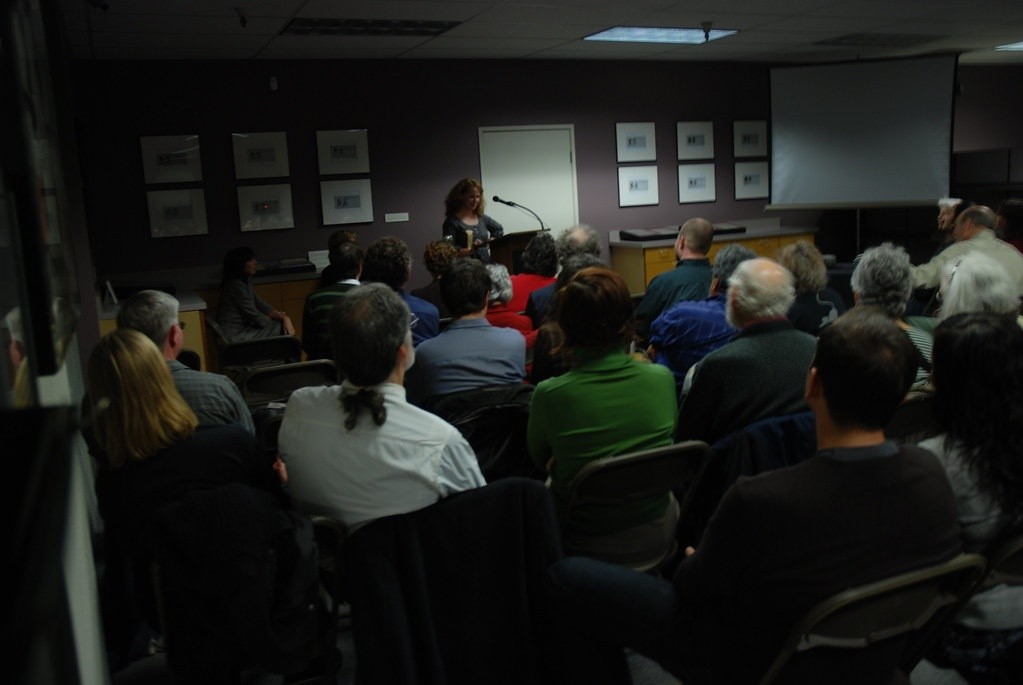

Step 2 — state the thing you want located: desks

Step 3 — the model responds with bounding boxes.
[612,225,825,305]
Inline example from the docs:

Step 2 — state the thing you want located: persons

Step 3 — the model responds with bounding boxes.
[4,305,26,368]
[78,290,345,685]
[302,194,1023,565]
[909,312,1023,674]
[442,177,503,266]
[545,311,964,684]
[216,246,295,372]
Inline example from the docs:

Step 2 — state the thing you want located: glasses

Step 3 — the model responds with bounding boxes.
[178,321,186,332]
[408,311,421,332]
[948,222,967,229]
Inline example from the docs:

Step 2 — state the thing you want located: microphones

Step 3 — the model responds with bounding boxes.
[493,195,514,206]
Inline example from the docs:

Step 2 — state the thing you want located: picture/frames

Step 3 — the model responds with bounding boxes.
[616,120,769,207]
[137,126,376,238]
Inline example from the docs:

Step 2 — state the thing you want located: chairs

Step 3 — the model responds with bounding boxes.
[241,357,346,406]
[425,377,539,476]
[205,309,306,378]
[684,407,820,539]
[888,393,951,445]
[742,541,996,685]
[954,510,1023,634]
[566,434,712,576]
[331,476,576,685]
